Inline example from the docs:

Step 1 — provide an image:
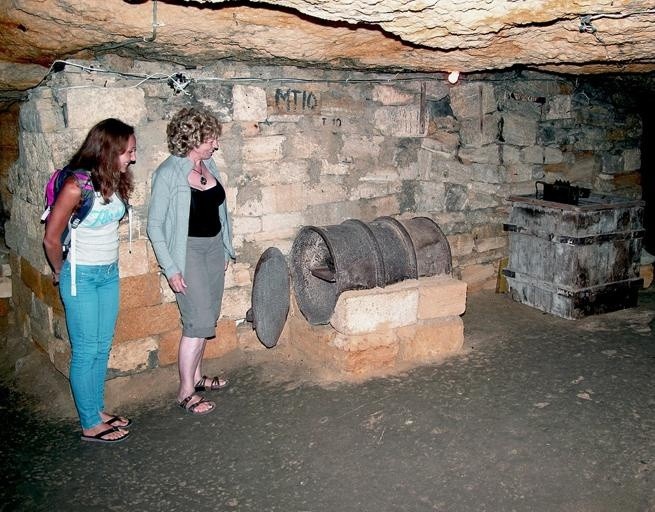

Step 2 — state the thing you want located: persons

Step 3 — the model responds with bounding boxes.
[42,119,136,444]
[147,109,237,415]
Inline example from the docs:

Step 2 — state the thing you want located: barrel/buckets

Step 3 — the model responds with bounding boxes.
[287,216,453,326]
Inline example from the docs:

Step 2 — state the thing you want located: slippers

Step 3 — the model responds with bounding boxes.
[81,414,133,443]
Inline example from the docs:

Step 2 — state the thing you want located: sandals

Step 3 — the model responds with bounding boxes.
[176,375,228,414]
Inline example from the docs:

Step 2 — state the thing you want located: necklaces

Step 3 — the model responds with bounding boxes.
[193,163,207,185]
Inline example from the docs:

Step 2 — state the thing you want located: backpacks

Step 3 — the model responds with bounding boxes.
[41,169,71,257]
